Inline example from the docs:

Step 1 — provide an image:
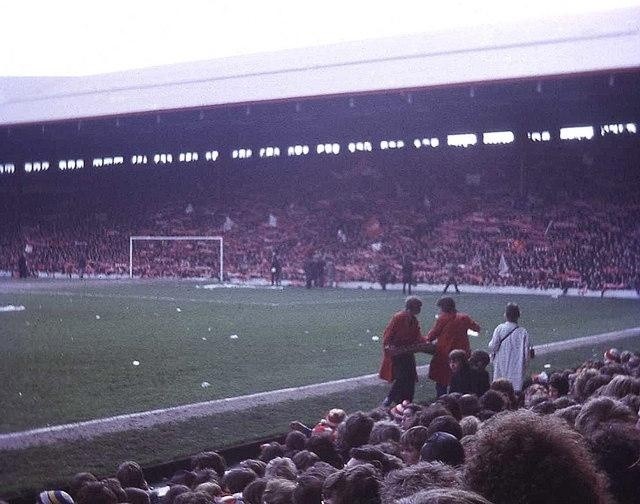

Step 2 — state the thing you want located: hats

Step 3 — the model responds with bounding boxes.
[388,400,411,419]
[326,408,349,427]
[603,348,621,365]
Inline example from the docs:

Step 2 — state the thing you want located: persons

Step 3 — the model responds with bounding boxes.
[488,301,531,395]
[379,295,433,407]
[1,189,640,301]
[425,297,481,397]
[1,346,639,502]
